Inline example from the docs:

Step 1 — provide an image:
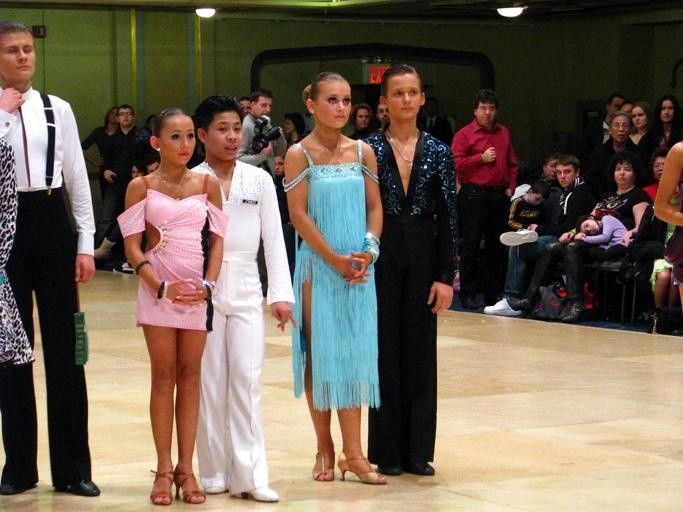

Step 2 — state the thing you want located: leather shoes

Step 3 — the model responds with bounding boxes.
[401,457,436,476]
[203,487,228,494]
[54,478,100,497]
[377,462,403,476]
[0,481,37,495]
[233,485,280,502]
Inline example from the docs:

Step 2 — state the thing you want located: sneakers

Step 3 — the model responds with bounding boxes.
[483,297,523,317]
[499,228,539,247]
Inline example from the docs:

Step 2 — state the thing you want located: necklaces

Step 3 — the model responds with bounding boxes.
[159,171,184,195]
[362,65,460,475]
[387,130,413,169]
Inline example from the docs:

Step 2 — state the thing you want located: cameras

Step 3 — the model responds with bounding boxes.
[252,115,281,153]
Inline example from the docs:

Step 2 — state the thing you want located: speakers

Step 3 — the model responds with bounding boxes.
[32,25,45,38]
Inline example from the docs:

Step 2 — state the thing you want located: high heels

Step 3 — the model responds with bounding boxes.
[337,450,388,485]
[561,301,590,323]
[173,464,206,505]
[506,296,536,318]
[312,451,336,482]
[150,463,174,506]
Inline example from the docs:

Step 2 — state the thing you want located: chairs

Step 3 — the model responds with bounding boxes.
[528,254,683,327]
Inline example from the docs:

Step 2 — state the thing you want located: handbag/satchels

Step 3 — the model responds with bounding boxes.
[648,309,682,335]
[538,283,572,321]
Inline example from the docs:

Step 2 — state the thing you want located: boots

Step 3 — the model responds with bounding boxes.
[94,237,116,261]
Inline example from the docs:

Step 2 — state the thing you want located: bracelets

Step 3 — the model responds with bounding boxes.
[203,281,216,332]
[362,233,381,264]
[157,281,169,300]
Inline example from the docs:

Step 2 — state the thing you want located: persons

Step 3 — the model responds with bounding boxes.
[189,95,296,503]
[452,89,683,334]
[116,106,230,506]
[284,72,388,485]
[349,101,388,140]
[0,20,101,497]
[81,105,160,272]
[236,90,307,296]
[0,134,36,370]
